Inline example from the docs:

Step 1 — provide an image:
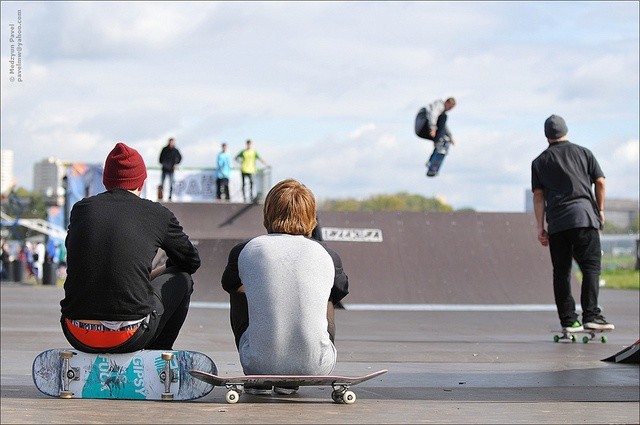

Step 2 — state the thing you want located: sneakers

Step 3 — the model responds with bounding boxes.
[272,386,298,397]
[241,388,273,394]
[583,315,616,333]
[562,318,583,333]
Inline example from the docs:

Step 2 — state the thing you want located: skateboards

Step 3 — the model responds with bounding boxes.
[552,328,611,345]
[187,367,388,404]
[426,144,449,178]
[30,348,219,403]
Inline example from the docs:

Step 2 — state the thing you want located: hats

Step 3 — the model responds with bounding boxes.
[544,114,568,137]
[102,139,148,192]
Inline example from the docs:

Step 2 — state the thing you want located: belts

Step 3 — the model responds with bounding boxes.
[62,314,150,351]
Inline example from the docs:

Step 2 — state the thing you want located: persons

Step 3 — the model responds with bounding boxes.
[415,98,457,167]
[59,142,202,354]
[531,115,615,332]
[216,142,232,199]
[0,235,66,287]
[159,138,182,199]
[234,140,268,202]
[221,179,349,395]
